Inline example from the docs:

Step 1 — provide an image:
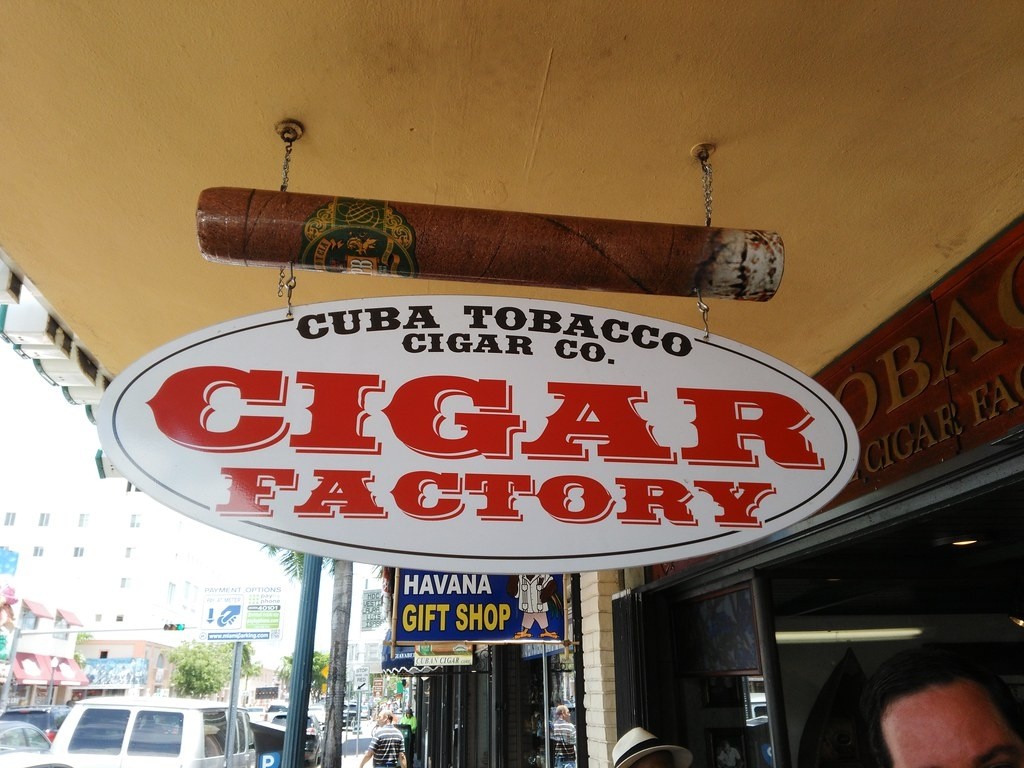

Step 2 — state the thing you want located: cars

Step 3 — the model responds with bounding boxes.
[0,691,372,768]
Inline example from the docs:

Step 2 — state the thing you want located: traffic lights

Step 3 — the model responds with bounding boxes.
[163,623,185,632]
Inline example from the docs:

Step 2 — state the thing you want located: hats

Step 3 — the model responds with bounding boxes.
[405,708,413,713]
[612,727,693,768]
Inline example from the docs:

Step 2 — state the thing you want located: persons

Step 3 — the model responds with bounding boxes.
[360,709,406,768]
[859,642,1024,768]
[400,709,418,763]
[611,727,692,768]
[550,705,577,768]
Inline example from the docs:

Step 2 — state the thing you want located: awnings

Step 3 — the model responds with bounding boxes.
[381,629,443,674]
[10,652,89,685]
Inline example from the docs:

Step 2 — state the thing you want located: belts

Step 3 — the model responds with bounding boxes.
[376,764,396,767]
